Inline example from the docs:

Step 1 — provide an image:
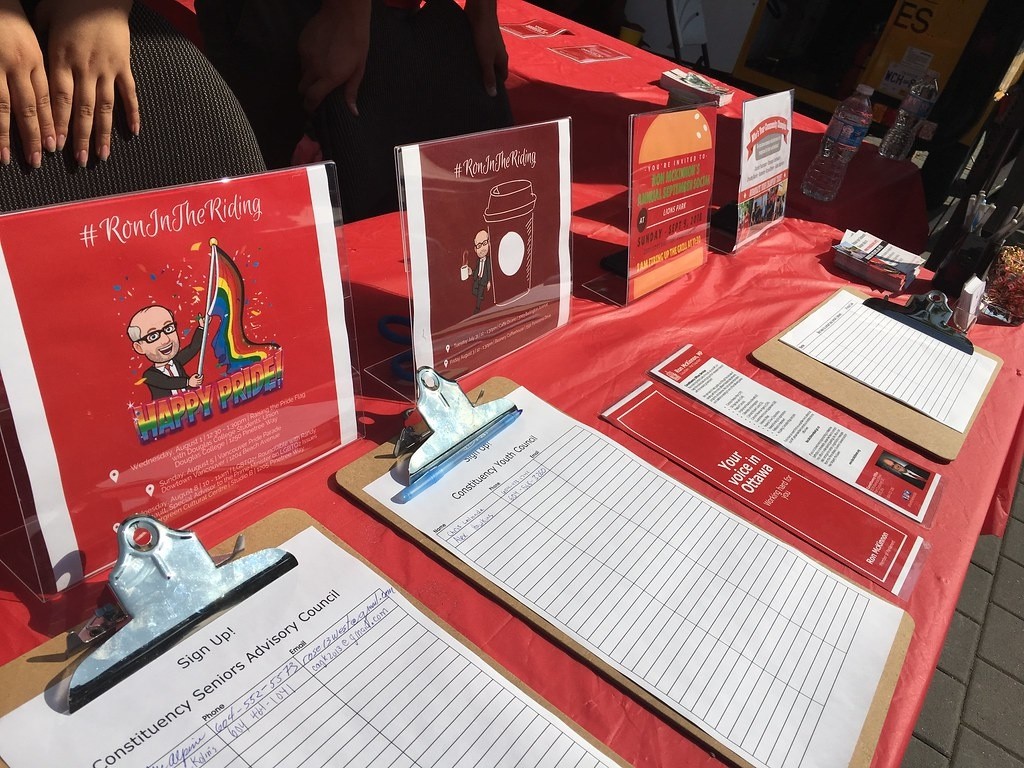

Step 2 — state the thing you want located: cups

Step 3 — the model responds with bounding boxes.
[931,227,1007,295]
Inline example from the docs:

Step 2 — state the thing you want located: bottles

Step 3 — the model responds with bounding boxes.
[877,68,939,160]
[800,82,874,202]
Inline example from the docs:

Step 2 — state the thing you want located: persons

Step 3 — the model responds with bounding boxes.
[0,0,266,217]
[193,0,517,223]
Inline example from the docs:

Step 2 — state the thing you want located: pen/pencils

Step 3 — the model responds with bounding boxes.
[965,189,1024,248]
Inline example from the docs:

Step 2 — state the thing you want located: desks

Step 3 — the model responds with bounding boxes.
[0,0,1023,768]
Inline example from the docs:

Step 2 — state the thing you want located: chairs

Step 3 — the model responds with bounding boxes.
[664,1,712,77]
[317,1,516,224]
[0,0,268,214]
